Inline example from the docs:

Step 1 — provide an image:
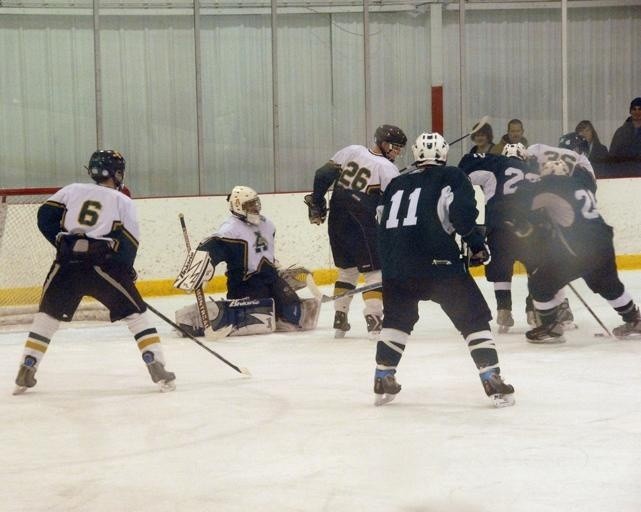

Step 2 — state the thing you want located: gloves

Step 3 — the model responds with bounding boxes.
[471,240,491,267]
[304,195,326,225]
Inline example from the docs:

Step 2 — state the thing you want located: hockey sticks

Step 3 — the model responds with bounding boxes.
[306,274,383,303]
[179,213,234,341]
[567,282,641,341]
[143,299,252,379]
[398,114,489,173]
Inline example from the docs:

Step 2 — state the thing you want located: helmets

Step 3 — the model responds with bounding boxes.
[374,125,407,162]
[413,131,449,166]
[229,186,262,226]
[89,149,125,183]
[502,132,588,177]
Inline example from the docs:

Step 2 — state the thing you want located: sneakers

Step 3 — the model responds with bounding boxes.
[15,364,36,387]
[333,310,351,331]
[497,298,574,340]
[367,315,382,332]
[148,363,174,383]
[613,321,641,335]
[375,371,400,394]
[483,374,514,396]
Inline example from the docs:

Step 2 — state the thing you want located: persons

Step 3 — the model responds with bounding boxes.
[605,95,640,177]
[11,148,177,388]
[463,120,497,158]
[525,130,599,326]
[575,118,611,178]
[303,124,409,332]
[457,139,540,329]
[174,185,320,339]
[513,159,641,341]
[488,118,532,158]
[372,130,516,399]
